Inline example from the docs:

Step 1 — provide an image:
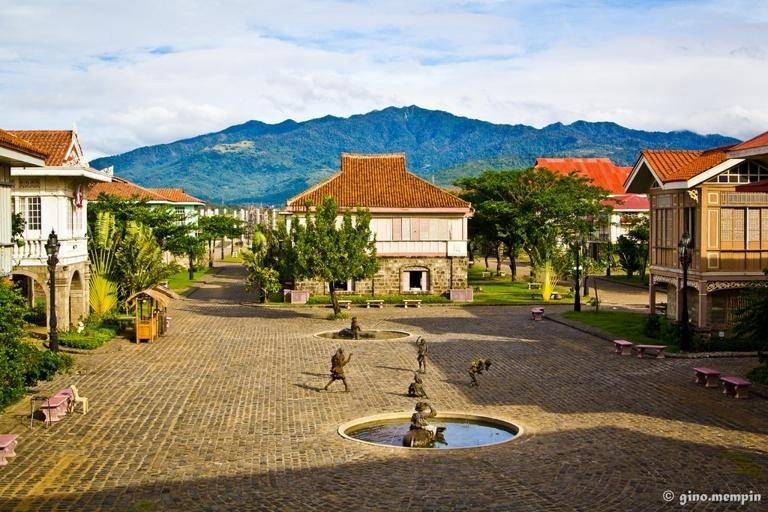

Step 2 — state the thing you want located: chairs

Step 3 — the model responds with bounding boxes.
[70,384,90,415]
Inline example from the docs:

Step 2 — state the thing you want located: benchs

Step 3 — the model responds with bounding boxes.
[54,387,73,412]
[634,345,669,358]
[528,283,542,289]
[0,433,20,465]
[614,339,633,356]
[365,299,385,309]
[337,299,353,309]
[403,299,423,308]
[41,396,71,422]
[532,307,545,321]
[719,376,752,398]
[694,366,721,387]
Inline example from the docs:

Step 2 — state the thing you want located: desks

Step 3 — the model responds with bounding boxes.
[29,393,52,430]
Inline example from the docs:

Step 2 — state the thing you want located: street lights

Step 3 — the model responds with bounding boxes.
[568,231,587,313]
[43,229,65,354]
[673,234,694,333]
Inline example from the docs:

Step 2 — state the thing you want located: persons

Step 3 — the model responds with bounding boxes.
[323,315,492,429]
